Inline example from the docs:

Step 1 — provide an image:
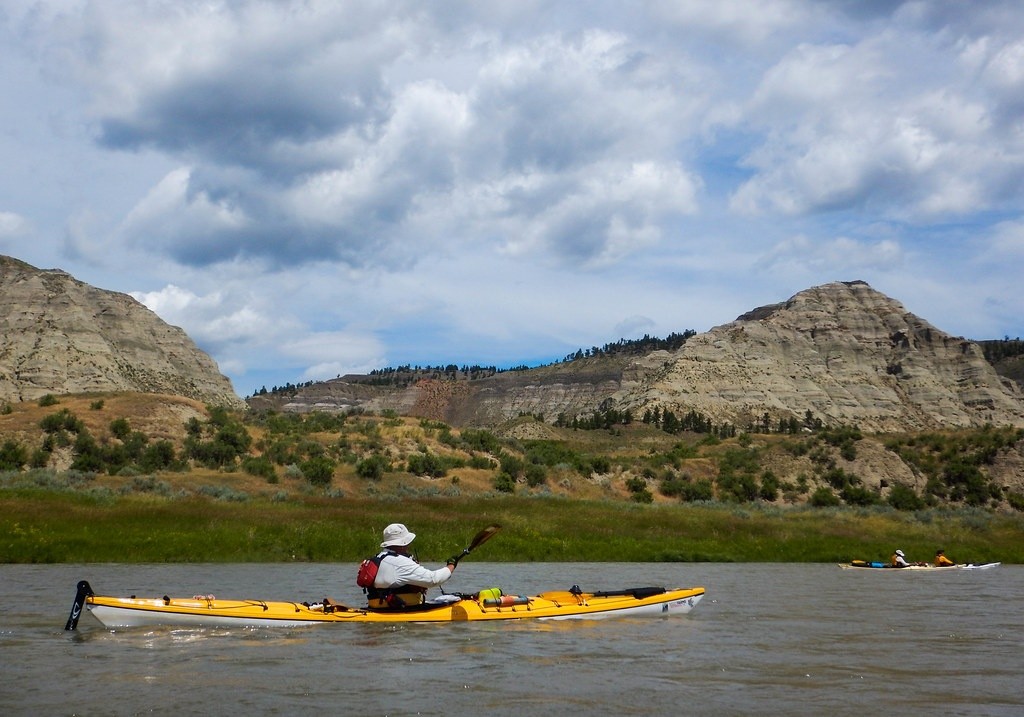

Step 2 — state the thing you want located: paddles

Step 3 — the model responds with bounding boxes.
[482,596,593,610]
[458,522,503,560]
[540,586,667,601]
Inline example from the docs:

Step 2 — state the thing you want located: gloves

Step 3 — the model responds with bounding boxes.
[446,556,458,569]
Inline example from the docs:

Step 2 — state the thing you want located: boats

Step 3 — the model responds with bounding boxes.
[67,581,707,634]
[836,562,1002,571]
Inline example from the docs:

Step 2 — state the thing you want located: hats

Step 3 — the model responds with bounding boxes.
[895,549,904,556]
[380,523,417,548]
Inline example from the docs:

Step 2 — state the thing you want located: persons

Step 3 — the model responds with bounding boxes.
[935,550,955,566]
[892,549,918,568]
[356,523,463,613]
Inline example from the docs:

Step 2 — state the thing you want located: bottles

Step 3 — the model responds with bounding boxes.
[479,588,502,602]
